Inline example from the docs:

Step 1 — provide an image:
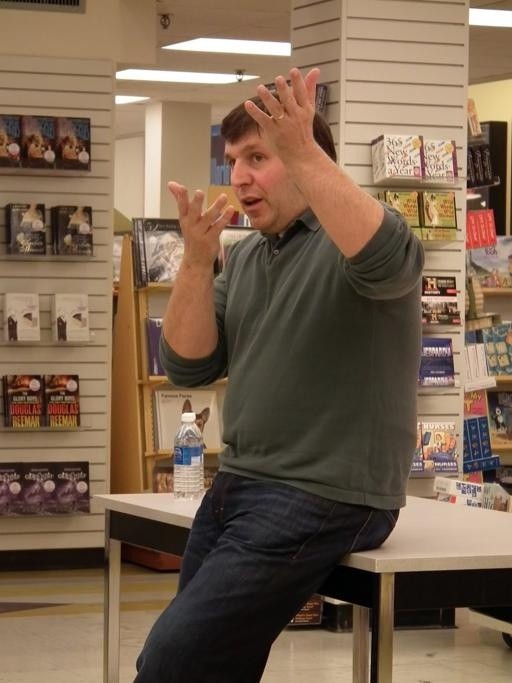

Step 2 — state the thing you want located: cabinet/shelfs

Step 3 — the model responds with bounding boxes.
[379,177,461,481]
[1,155,101,519]
[112,236,230,570]
[464,237,511,488]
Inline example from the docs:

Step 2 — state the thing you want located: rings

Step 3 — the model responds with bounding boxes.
[274,110,285,121]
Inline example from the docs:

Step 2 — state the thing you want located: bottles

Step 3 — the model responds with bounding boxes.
[171,410,207,502]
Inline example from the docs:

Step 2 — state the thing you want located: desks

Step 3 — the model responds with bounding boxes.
[90,488,512,683]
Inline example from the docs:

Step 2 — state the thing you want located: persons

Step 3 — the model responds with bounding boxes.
[134,66,426,683]
